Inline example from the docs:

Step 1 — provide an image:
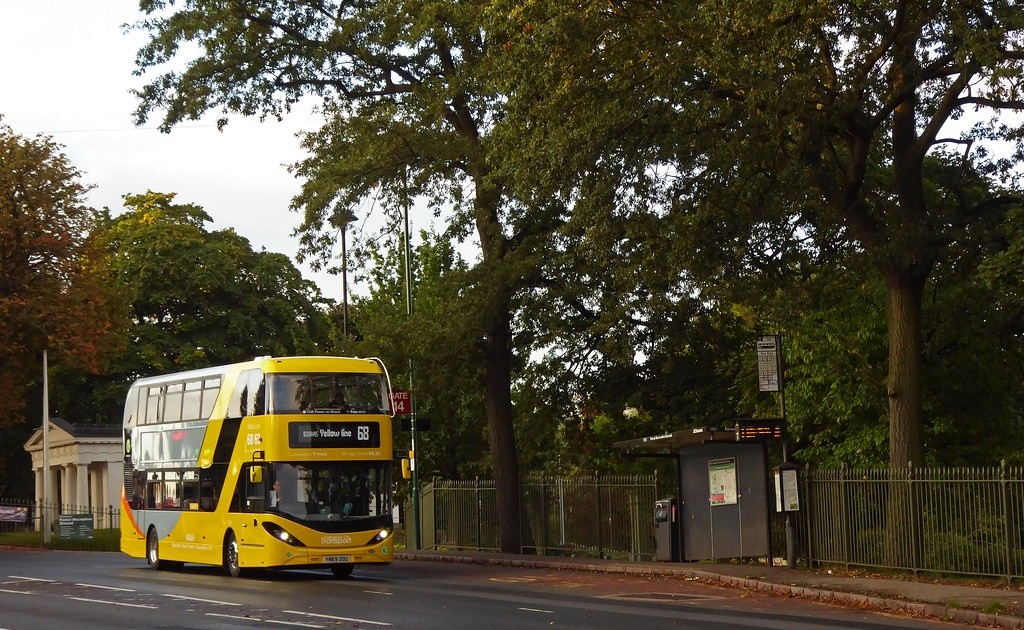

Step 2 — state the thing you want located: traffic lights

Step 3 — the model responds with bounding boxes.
[400,419,411,431]
[417,419,431,431]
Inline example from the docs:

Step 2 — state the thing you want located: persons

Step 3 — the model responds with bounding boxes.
[270,479,290,510]
[329,392,351,414]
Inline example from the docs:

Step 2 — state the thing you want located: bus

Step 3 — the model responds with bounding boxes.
[120,356,414,578]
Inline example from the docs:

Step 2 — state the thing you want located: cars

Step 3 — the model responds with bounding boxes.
[164,498,178,507]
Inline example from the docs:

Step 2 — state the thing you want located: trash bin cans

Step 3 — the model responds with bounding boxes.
[654,498,679,562]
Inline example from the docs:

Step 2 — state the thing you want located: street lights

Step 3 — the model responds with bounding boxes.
[328,213,358,336]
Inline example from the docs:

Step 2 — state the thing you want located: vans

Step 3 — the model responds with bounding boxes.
[175,481,214,509]
